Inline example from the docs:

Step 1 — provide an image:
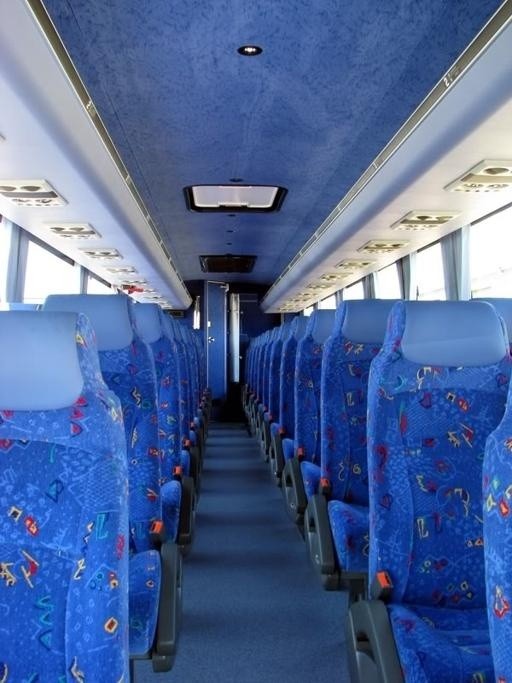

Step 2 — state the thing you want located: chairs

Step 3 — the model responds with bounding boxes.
[239,298,512,511]
[41,293,185,675]
[483,380,512,681]
[2,312,132,683]
[0,295,214,551]
[346,299,512,678]
[306,296,400,590]
